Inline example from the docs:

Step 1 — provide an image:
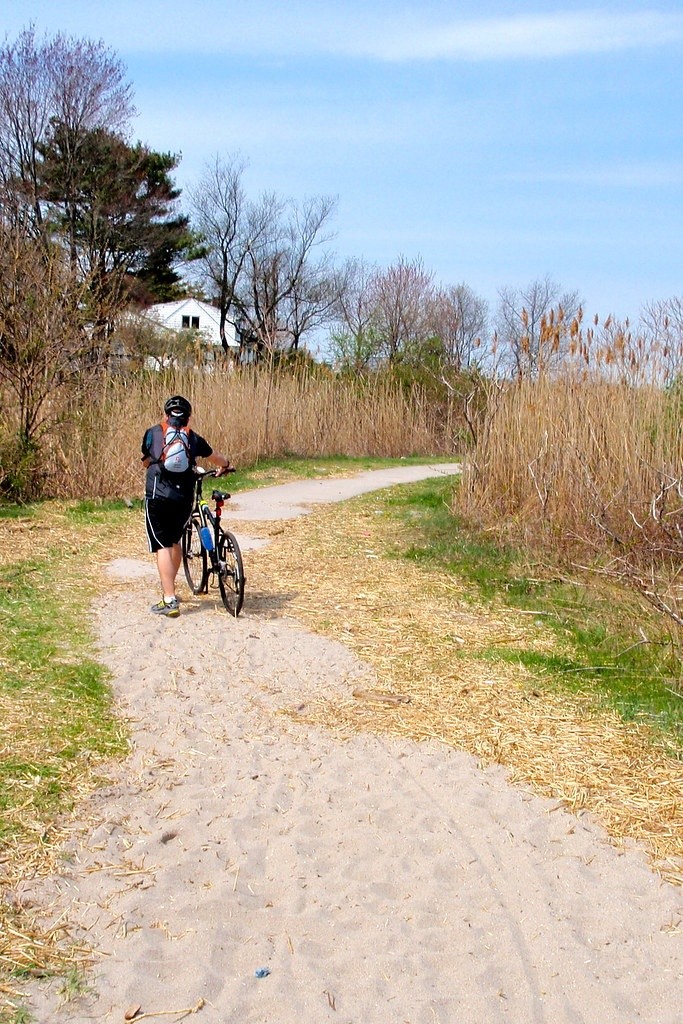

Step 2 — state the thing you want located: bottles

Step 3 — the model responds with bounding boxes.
[201,525,213,550]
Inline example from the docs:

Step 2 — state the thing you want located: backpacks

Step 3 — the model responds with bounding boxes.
[159,422,190,472]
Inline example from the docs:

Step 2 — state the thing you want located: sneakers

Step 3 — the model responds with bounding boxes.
[152,600,180,617]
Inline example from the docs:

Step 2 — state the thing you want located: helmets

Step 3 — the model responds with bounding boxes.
[165,396,192,417]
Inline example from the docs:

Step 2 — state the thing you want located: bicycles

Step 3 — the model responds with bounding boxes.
[181,465,250,616]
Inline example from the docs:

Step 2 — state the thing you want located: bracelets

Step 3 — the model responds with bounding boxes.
[222,460,230,468]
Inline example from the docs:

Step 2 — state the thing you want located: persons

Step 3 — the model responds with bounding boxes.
[140,396,232,619]
